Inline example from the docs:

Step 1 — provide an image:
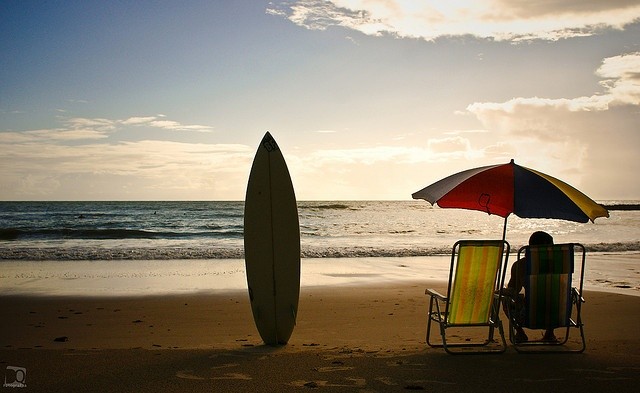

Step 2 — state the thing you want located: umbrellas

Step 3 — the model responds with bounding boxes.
[411,158,610,291]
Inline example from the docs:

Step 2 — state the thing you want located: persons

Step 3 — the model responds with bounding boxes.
[493,231,566,343]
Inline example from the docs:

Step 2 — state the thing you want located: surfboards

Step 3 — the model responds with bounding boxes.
[244,131,300,345]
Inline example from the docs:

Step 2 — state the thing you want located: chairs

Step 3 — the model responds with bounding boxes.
[425,240,510,356]
[494,243,587,354]
